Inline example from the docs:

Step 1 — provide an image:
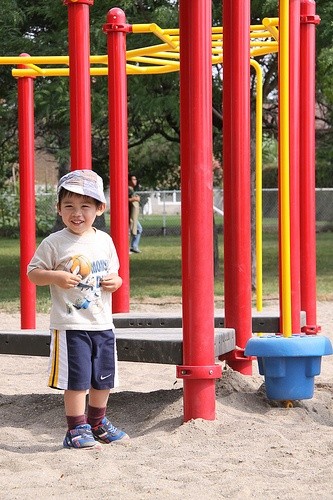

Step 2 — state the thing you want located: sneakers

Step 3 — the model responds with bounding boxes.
[63,424,100,449]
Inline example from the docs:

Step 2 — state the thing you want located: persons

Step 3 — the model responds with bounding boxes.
[27,170,129,447]
[128,174,142,253]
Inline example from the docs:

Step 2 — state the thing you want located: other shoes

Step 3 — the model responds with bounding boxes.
[129,249,140,253]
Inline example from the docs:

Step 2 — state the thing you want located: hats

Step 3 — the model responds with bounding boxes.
[57,169,106,204]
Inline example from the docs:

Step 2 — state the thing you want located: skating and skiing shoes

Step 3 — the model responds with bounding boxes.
[93,418,129,444]
